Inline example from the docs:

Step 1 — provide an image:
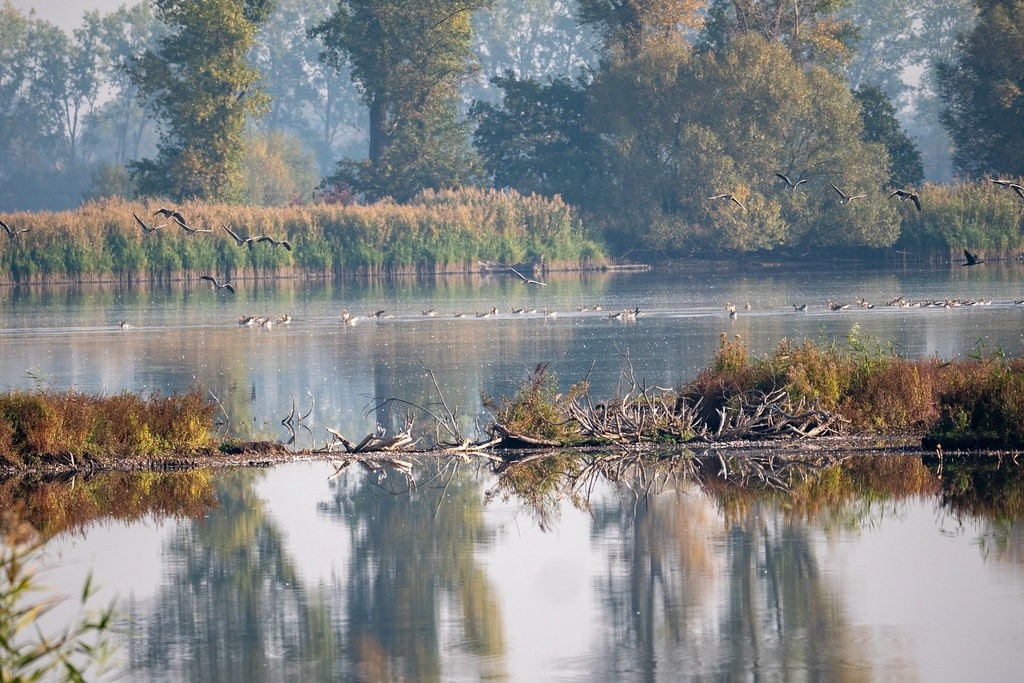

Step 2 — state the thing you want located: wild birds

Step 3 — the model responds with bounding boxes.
[776,172,807,190]
[202,276,235,294]
[422,309,438,316]
[725,295,997,321]
[338,309,359,324]
[120,321,128,328]
[450,312,465,318]
[577,304,640,319]
[0,220,30,239]
[962,249,985,267]
[239,314,291,328]
[1015,299,1024,304]
[222,225,292,251]
[831,184,867,205]
[368,311,396,318]
[544,308,557,317]
[511,307,537,314]
[475,307,499,318]
[708,194,744,211]
[989,179,1024,191]
[889,189,921,211]
[133,208,214,235]
[510,268,548,286]
